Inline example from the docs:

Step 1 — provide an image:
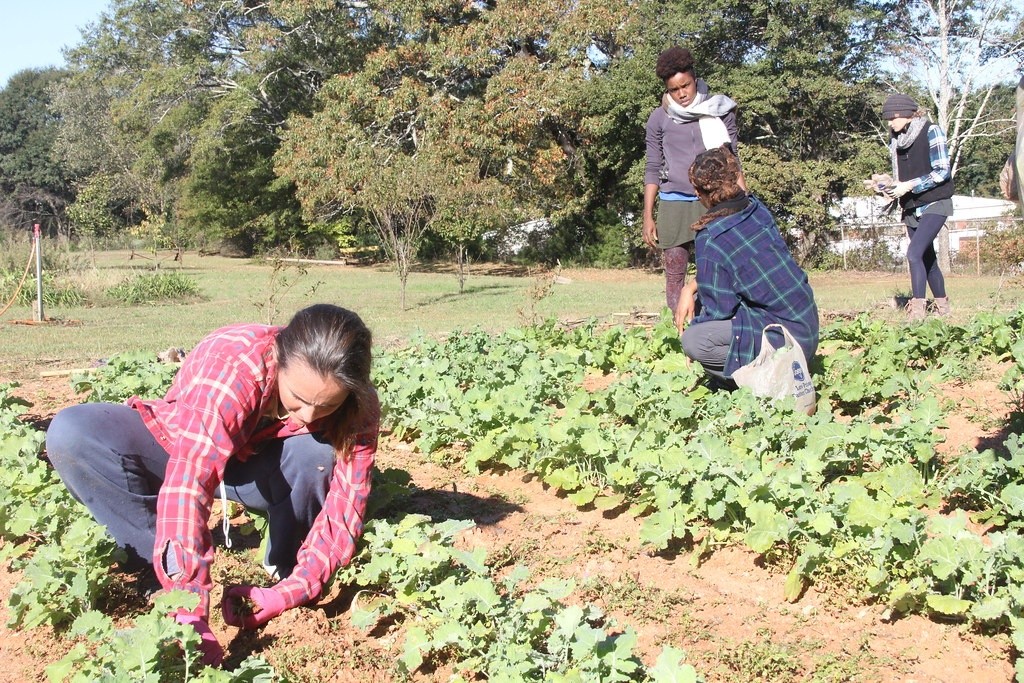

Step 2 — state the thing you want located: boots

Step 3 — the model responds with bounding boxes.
[905,297,926,326]
[927,296,950,318]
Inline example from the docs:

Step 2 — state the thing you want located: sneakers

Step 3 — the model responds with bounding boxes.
[138,568,163,601]
[262,559,298,581]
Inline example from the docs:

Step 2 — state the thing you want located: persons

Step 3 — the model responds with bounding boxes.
[879,93,955,327]
[45,305,381,666]
[673,146,818,392]
[641,49,740,350]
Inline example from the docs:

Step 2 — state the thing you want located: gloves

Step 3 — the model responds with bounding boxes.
[172,614,223,667]
[221,583,286,629]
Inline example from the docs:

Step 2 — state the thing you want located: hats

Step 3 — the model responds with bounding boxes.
[883,93,917,120]
